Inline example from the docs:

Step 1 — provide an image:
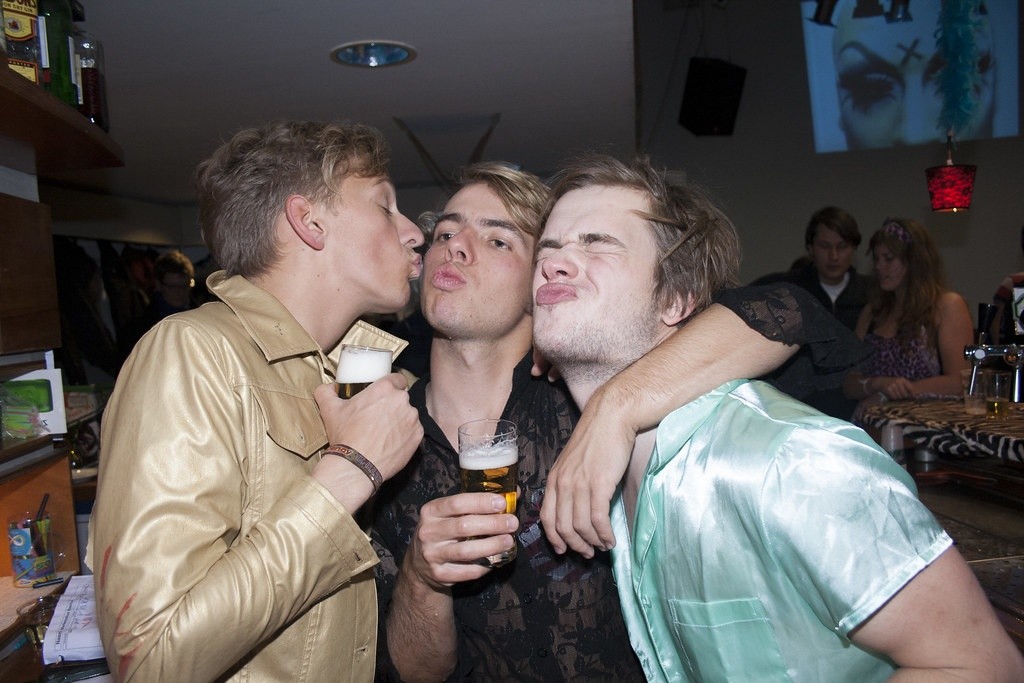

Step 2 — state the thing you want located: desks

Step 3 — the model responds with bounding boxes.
[855,394,1024,502]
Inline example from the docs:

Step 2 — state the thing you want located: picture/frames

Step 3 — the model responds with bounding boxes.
[0,351,68,434]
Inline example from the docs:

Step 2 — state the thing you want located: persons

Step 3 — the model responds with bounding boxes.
[833,0,999,149]
[750,206,872,333]
[989,270,1024,345]
[841,218,974,423]
[131,244,208,346]
[531,155,1024,683]
[89,121,425,683]
[364,161,877,683]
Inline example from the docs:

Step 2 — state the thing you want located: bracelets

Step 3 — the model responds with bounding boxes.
[321,444,383,497]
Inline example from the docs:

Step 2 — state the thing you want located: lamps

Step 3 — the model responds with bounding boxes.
[925,129,977,213]
[810,0,840,27]
[885,0,912,22]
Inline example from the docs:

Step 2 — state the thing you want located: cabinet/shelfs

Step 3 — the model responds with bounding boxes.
[0,63,125,683]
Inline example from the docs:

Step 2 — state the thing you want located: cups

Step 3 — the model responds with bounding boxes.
[983,370,1012,420]
[960,369,990,415]
[458,418,518,560]
[15,595,59,655]
[8,511,56,588]
[882,425,907,471]
[335,345,392,398]
